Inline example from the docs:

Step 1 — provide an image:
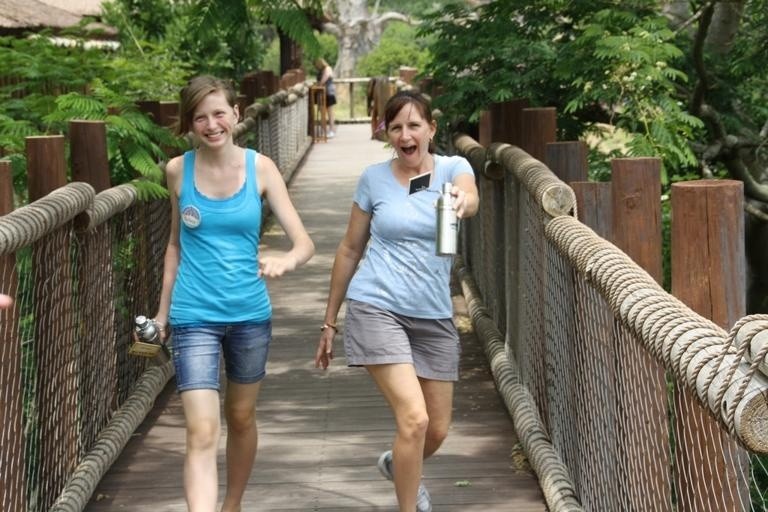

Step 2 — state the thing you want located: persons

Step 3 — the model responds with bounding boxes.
[313,89,479,512]
[132,74,315,512]
[314,57,336,138]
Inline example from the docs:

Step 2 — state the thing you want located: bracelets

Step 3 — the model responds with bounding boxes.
[320,323,339,334]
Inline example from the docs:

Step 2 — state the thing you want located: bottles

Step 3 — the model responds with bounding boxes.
[135,315,170,367]
[435,182,457,257]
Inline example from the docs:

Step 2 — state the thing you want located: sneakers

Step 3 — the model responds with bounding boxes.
[319,130,335,137]
[377,450,432,512]
